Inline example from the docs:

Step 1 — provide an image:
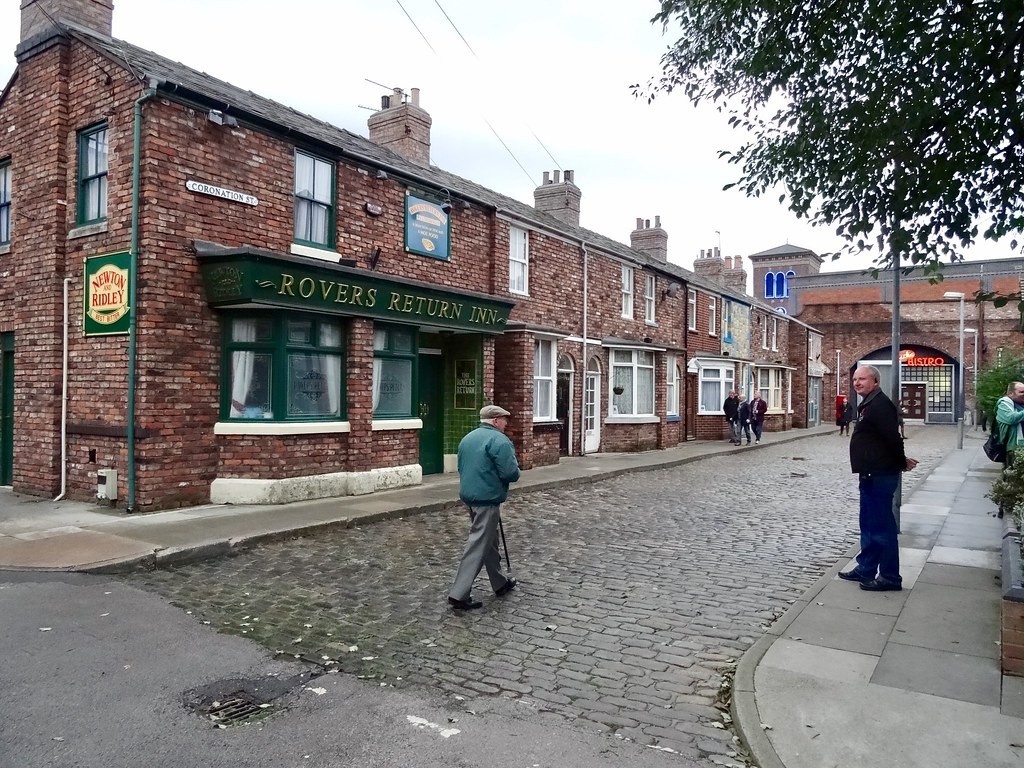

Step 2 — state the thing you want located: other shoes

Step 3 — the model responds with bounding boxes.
[839,432,843,435]
[756,440,759,443]
[746,442,750,446]
[735,442,741,446]
[846,433,849,436]
[729,439,735,443]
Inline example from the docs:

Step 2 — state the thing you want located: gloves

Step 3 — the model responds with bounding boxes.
[730,418,732,421]
[746,419,750,423]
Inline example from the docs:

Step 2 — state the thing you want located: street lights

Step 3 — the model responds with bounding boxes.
[943,291,965,447]
[836,348,842,394]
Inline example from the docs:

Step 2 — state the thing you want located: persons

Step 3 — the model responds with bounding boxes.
[976,403,989,433]
[897,400,908,440]
[445,404,520,609]
[749,391,767,444]
[837,366,919,591]
[995,382,1024,470]
[837,398,853,436]
[723,391,752,446]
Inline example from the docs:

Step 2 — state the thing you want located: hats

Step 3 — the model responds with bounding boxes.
[480,405,510,419]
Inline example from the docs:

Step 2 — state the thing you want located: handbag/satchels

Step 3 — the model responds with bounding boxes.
[983,416,1011,462]
[836,419,846,425]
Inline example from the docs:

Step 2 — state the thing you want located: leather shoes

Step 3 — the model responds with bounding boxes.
[495,577,516,596]
[838,571,875,583]
[448,597,482,608]
[860,579,902,592]
